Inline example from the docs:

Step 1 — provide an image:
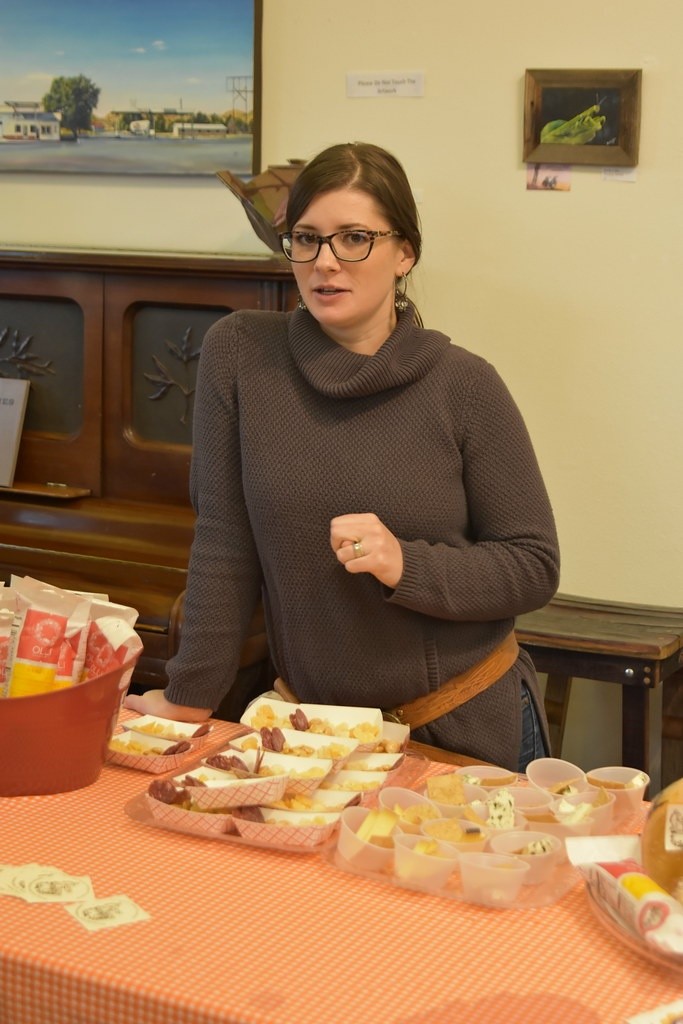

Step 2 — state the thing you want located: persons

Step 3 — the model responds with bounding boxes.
[123,140,561,776]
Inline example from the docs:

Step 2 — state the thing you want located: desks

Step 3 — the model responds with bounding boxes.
[0,700,683,1024]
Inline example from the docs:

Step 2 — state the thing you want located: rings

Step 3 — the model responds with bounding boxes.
[353,544,362,558]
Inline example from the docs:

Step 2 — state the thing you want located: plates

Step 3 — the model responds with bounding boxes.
[585,883,683,972]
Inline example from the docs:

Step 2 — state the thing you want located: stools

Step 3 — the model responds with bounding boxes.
[514,591,683,802]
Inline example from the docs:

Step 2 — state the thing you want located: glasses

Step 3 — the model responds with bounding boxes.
[278,229,402,262]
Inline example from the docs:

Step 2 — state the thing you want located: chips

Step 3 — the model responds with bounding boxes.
[359,774,628,860]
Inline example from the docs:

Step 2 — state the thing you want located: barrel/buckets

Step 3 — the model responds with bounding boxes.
[0,648,143,796]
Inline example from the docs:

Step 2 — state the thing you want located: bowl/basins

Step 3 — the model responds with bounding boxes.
[336,756,653,905]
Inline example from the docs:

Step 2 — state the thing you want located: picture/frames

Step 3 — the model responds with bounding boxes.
[522,68,642,168]
[0,0,264,177]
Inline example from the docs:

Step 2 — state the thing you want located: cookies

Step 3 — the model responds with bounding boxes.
[147,708,308,802]
